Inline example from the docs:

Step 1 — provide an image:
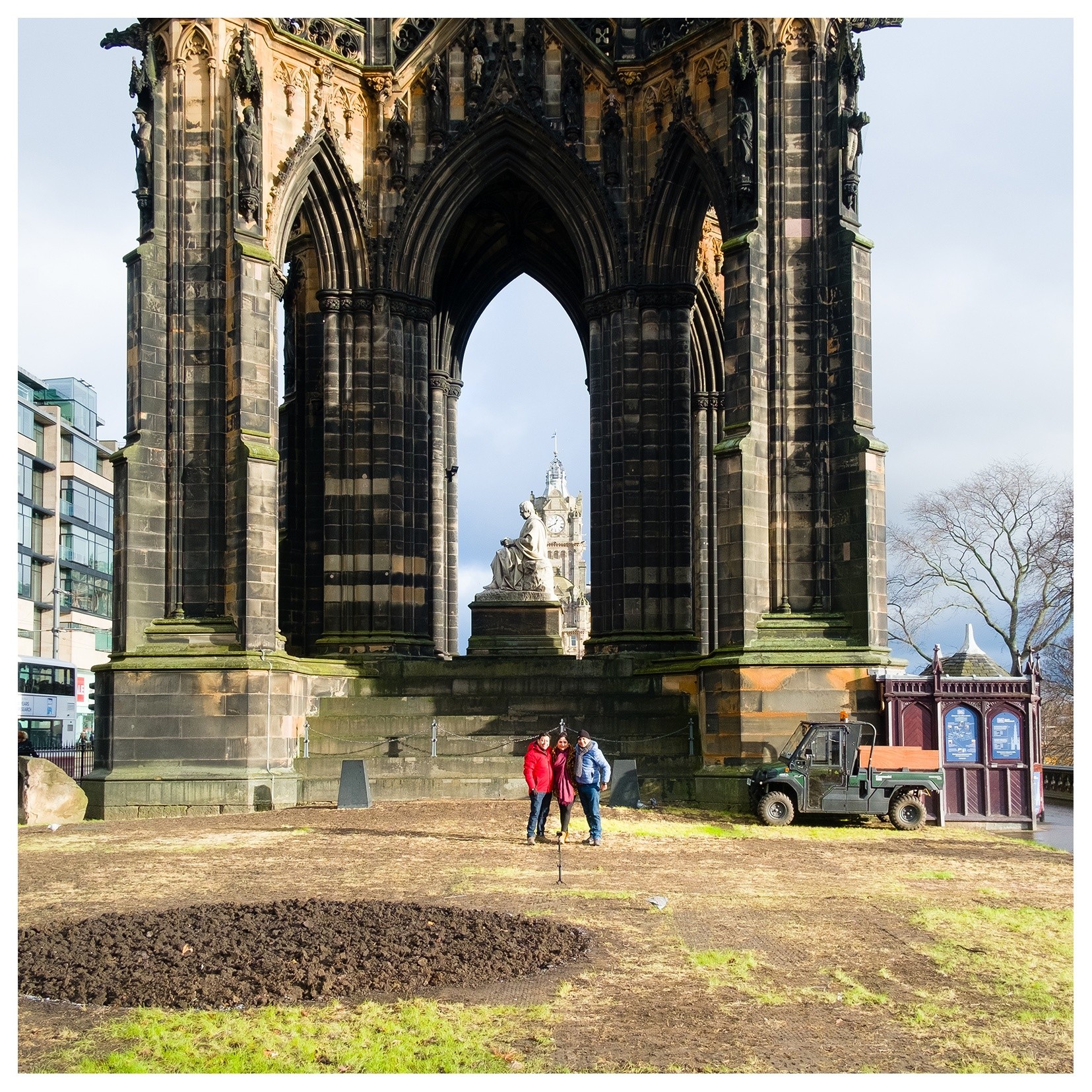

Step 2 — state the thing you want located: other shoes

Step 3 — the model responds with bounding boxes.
[587,837,602,845]
[558,831,570,845]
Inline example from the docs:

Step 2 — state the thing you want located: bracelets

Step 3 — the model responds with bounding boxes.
[531,788,534,790]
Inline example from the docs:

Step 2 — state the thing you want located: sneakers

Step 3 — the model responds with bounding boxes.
[535,836,551,843]
[527,835,536,845]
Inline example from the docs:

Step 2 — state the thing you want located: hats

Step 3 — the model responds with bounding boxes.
[577,729,591,739]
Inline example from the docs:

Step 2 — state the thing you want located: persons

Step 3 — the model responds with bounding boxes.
[78,729,87,745]
[549,731,576,845]
[483,498,549,592]
[522,733,556,846]
[18,730,39,758]
[573,729,612,846]
[89,730,95,751]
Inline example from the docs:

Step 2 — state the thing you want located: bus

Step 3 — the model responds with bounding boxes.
[17,655,77,750]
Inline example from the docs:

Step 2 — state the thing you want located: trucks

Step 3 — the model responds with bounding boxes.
[745,721,945,832]
